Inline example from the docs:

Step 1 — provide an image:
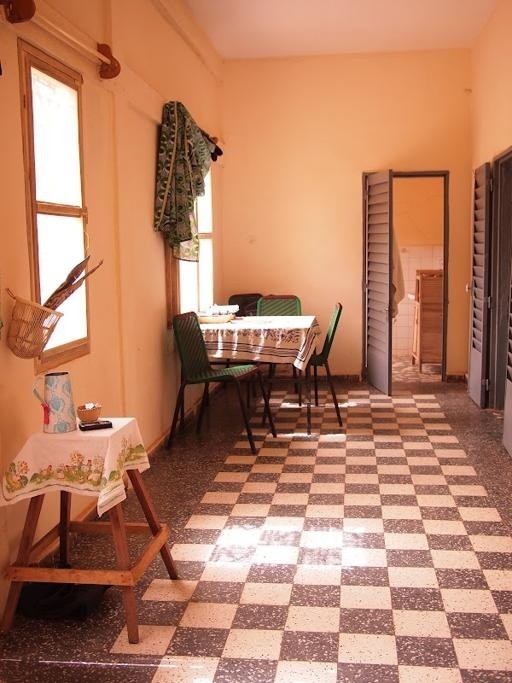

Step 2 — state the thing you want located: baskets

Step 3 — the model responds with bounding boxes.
[76,403,102,423]
[5,298,63,361]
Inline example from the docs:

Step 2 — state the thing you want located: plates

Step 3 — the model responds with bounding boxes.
[197,314,236,324]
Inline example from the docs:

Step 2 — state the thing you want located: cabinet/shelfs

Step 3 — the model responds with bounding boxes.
[411,268,443,372]
[0,417,179,645]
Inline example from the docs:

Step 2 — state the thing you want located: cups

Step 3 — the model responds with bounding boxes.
[78,403,101,422]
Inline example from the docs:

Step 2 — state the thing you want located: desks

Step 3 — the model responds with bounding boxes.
[199,315,323,434]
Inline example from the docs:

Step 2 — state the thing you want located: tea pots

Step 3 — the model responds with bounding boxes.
[32,369,77,431]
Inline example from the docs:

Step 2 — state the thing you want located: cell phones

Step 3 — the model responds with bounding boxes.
[79,420,112,431]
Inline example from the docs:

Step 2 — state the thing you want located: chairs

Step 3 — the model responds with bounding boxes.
[168,312,277,454]
[259,303,347,426]
[222,293,273,390]
[253,296,302,399]
